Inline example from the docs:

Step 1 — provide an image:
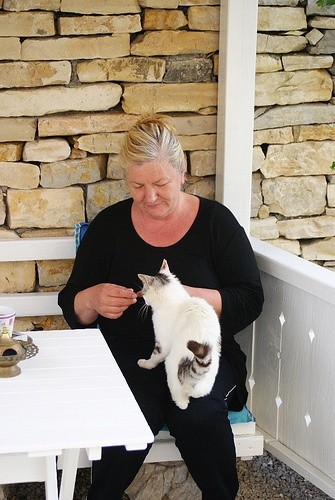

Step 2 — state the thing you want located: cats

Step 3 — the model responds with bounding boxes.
[136,257,223,411]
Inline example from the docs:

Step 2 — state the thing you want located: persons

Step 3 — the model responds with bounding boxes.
[56,111,266,500]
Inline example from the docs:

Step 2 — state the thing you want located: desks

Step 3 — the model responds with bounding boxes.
[0,328,154,500]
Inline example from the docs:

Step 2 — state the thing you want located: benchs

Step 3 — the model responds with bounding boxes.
[0,224,263,463]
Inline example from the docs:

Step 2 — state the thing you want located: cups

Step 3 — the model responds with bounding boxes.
[0,306,17,339]
